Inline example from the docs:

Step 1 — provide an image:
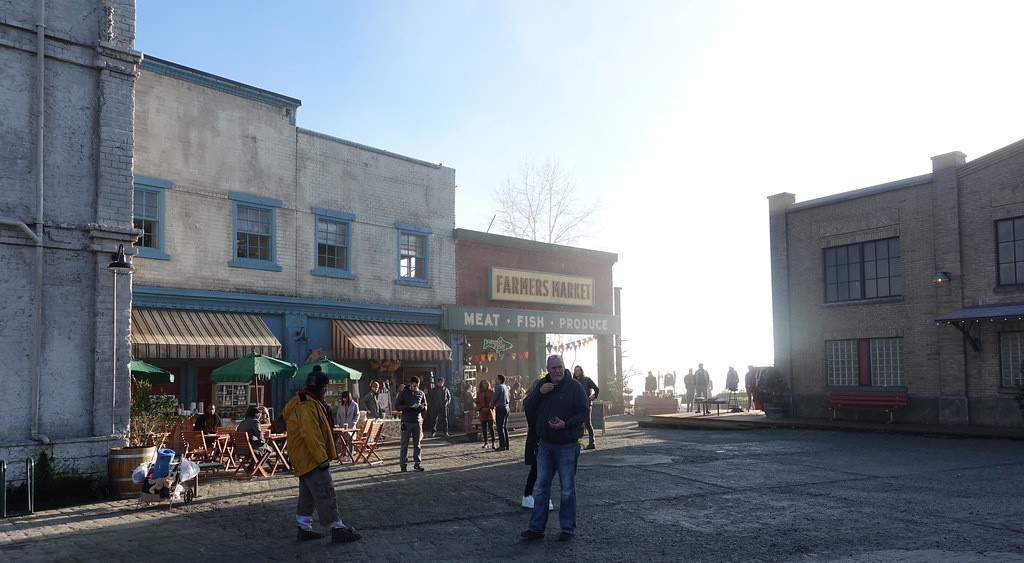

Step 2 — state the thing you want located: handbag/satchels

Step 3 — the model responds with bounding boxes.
[179,454,200,482]
[132,462,153,484]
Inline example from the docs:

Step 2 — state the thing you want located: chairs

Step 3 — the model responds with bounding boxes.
[155,415,387,481]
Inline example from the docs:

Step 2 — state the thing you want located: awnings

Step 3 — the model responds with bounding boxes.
[130,307,281,358]
[331,319,452,361]
[935,303,1024,353]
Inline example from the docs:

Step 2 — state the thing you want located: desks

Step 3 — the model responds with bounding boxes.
[694,401,730,416]
[263,433,291,476]
[332,428,362,465]
[190,432,234,476]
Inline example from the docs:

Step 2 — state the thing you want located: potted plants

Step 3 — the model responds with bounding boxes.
[758,366,791,420]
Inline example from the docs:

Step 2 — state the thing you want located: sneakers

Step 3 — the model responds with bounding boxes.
[522,495,534,508]
[549,498,554,510]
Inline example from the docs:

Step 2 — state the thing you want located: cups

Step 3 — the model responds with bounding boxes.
[343,423,348,430]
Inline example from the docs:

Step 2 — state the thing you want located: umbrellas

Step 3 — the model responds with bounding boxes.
[292,356,363,402]
[130,360,175,384]
[210,350,298,407]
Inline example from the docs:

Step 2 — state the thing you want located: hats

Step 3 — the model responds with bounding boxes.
[306,365,328,386]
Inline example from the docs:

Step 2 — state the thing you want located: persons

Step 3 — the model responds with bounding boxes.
[280,365,362,543]
[510,355,599,541]
[360,381,386,440]
[476,379,496,448]
[463,384,476,432]
[394,376,427,472]
[237,407,273,477]
[397,384,406,399]
[643,363,754,413]
[337,391,360,463]
[193,403,223,468]
[489,374,510,451]
[271,405,295,471]
[428,377,451,437]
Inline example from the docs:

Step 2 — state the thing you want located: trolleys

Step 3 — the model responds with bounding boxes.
[139,434,194,511]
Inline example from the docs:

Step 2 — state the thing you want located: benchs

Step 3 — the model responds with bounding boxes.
[819,385,909,424]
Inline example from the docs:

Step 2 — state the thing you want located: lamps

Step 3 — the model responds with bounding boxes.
[931,271,952,283]
[107,242,138,275]
[455,329,472,348]
[295,326,311,345]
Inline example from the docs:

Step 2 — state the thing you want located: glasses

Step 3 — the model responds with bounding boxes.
[548,355,563,362]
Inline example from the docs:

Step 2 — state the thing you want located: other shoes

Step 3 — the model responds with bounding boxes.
[506,447,509,449]
[297,526,325,541]
[706,410,710,413]
[495,447,505,450]
[559,532,572,540]
[331,526,362,541]
[521,530,545,538]
[401,467,407,471]
[431,432,435,437]
[585,444,595,449]
[695,409,700,413]
[492,446,495,448]
[414,466,424,471]
[482,444,489,448]
[445,432,449,436]
